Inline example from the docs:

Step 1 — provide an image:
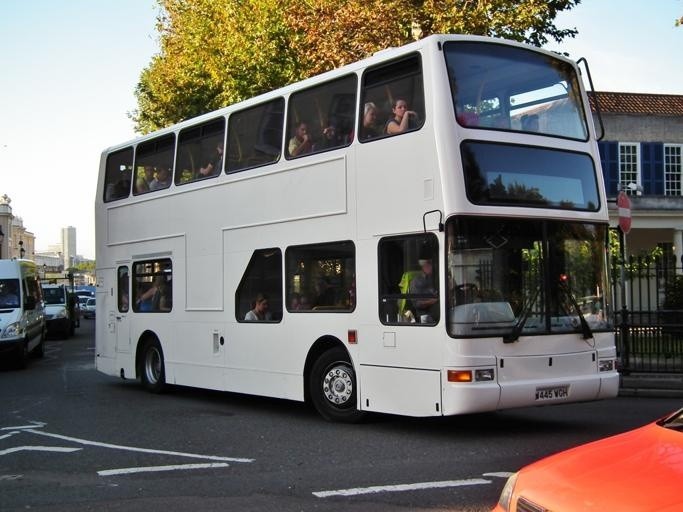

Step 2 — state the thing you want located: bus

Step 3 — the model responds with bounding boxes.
[94,33,621,425]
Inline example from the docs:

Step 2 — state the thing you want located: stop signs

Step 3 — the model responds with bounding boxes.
[618,191,632,233]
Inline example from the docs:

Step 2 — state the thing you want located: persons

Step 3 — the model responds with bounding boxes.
[492,114,511,129]
[117,266,173,312]
[318,121,348,152]
[361,100,382,140]
[244,292,275,322]
[0,283,18,306]
[520,114,541,132]
[451,97,480,128]
[112,168,136,198]
[287,275,355,313]
[406,254,464,327]
[383,98,420,135]
[134,162,156,195]
[198,139,225,179]
[286,119,315,158]
[148,158,172,191]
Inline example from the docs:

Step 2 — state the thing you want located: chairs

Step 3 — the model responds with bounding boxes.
[399,271,422,316]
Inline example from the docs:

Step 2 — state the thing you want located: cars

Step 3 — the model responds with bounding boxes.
[42,284,96,338]
[491,405,683,512]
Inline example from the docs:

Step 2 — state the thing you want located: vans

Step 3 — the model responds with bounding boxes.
[0,259,46,363]
